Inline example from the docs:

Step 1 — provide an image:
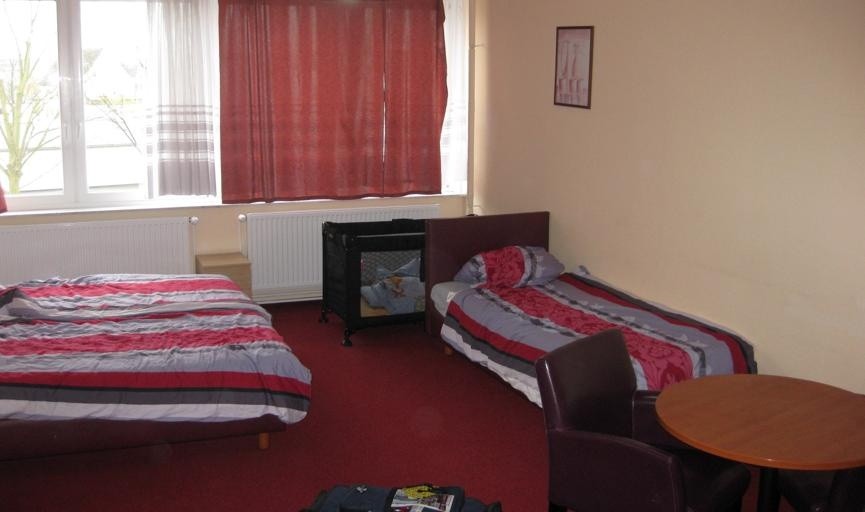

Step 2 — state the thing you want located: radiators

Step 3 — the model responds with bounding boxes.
[0,215,200,286]
[238,202,438,306]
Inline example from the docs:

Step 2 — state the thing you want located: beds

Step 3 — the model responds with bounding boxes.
[0,274,313,450]
[320,214,477,347]
[421,210,758,410]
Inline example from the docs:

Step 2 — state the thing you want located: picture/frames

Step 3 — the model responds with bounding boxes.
[554,25,594,108]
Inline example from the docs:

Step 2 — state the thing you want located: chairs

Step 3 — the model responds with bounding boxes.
[534,327,752,510]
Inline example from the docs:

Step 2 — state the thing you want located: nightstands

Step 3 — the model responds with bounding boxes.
[196,252,252,300]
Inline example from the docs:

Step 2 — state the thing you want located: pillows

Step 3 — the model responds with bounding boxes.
[454,247,566,287]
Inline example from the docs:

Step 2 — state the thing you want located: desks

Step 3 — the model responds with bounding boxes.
[655,374,865,512]
